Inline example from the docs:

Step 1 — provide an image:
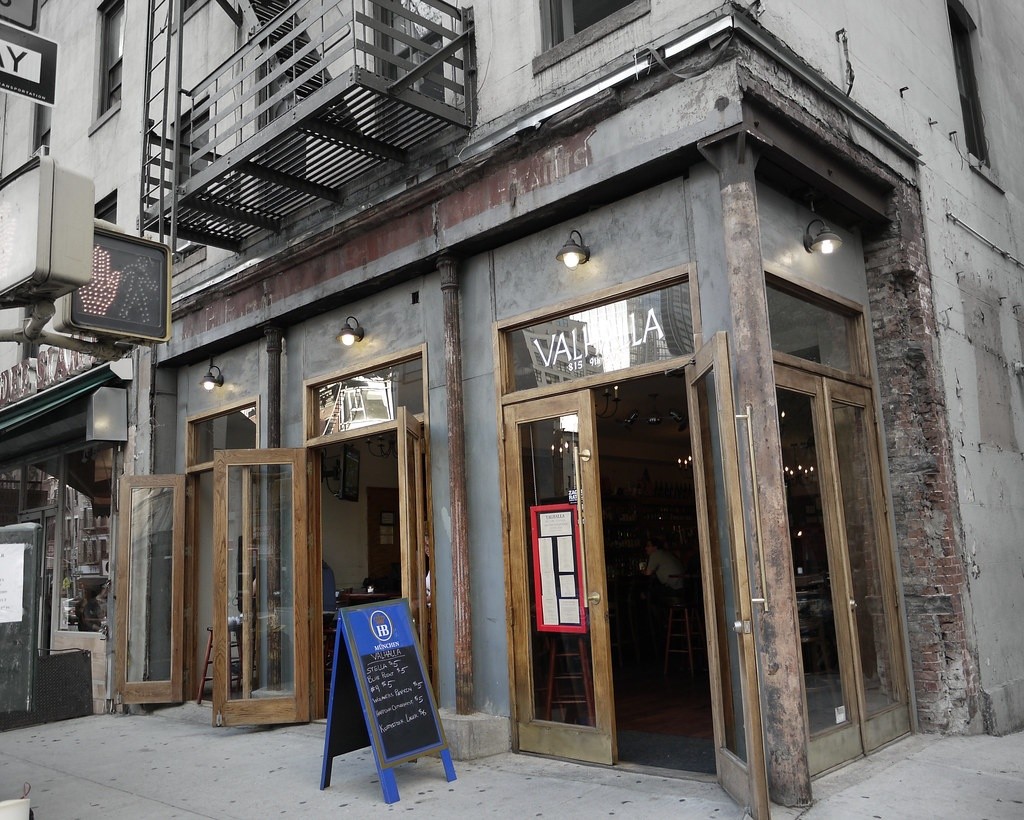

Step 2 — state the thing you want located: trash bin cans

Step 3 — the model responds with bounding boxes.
[324,598,450,770]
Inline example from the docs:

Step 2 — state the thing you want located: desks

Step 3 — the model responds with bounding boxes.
[338,590,403,605]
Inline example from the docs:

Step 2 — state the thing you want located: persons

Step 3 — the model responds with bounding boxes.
[323,560,336,624]
[633,537,684,604]
[253,544,279,595]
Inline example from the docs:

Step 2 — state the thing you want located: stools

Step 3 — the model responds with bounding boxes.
[661,599,707,678]
[195,623,255,705]
[796,575,840,719]
[544,634,594,727]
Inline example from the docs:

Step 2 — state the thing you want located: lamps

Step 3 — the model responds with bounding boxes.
[803,218,843,254]
[199,365,224,391]
[595,385,621,419]
[366,434,398,460]
[336,316,365,346]
[624,393,686,426]
[556,229,590,268]
